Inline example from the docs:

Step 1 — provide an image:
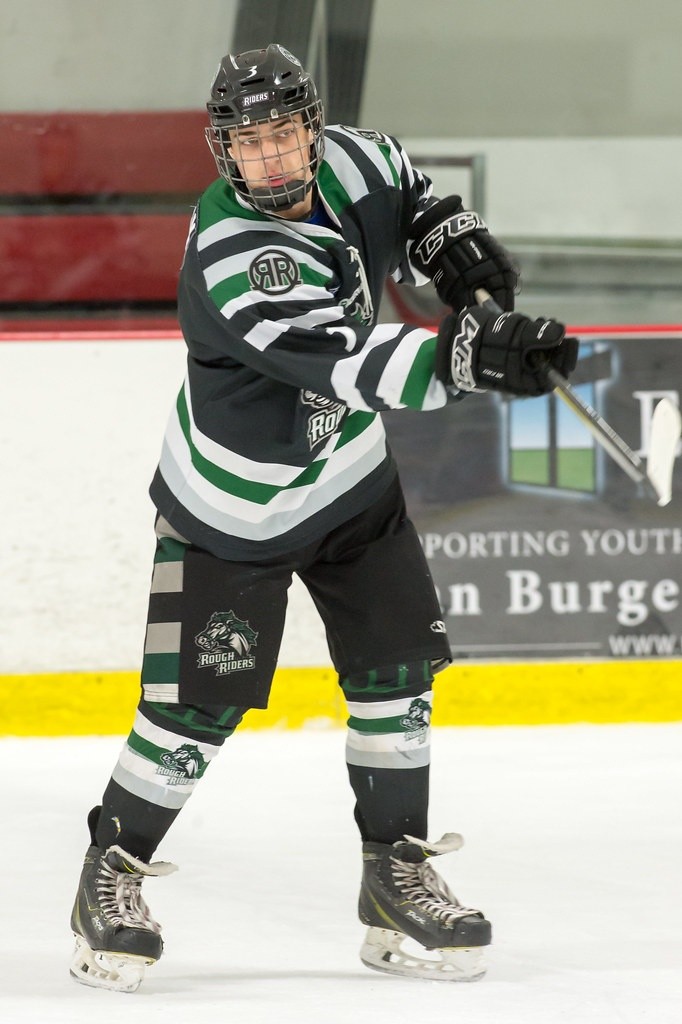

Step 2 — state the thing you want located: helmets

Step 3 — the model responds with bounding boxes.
[205,44,324,211]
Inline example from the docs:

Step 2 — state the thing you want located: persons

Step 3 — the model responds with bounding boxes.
[70,45,578,960]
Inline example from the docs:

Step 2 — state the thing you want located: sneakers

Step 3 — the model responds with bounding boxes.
[358,832,491,980]
[69,805,179,993]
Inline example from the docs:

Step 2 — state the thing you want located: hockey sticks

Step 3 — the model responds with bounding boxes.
[476,288,681,512]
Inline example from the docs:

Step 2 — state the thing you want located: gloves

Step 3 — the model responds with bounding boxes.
[435,306,579,397]
[408,194,520,312]
